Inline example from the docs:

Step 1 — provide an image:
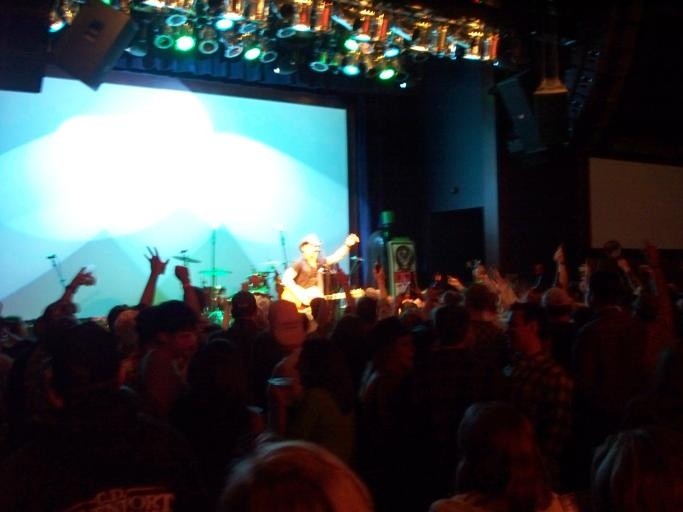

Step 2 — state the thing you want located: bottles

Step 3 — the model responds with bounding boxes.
[367,211,417,298]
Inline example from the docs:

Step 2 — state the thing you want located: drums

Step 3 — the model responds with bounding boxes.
[203,286,225,311]
[242,273,270,295]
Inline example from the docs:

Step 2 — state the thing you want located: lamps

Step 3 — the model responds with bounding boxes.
[46,0,570,95]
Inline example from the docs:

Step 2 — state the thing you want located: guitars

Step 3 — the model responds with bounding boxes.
[278,286,365,309]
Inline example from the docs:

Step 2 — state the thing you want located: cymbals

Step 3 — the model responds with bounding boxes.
[254,260,286,269]
[172,255,200,263]
[198,267,232,274]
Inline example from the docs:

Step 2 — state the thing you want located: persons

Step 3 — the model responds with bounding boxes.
[0,232,682,512]
